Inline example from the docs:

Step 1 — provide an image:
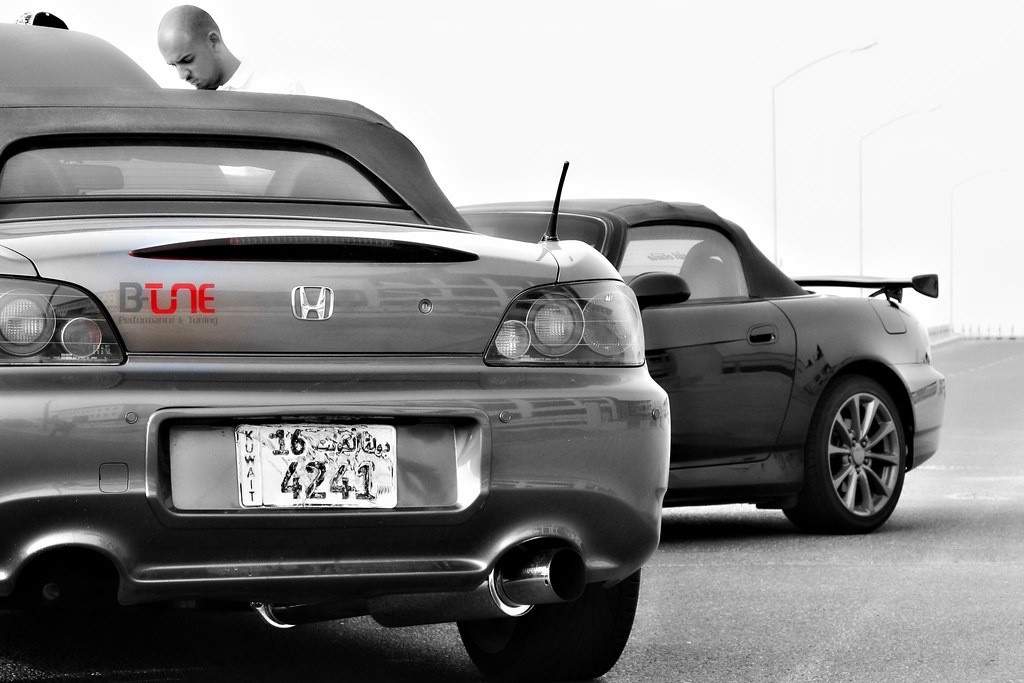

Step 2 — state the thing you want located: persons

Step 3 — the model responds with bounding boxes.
[153,4,292,197]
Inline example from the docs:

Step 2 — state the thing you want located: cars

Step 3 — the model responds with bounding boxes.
[461,200,947,536]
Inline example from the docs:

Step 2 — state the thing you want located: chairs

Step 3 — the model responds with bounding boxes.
[0,156,384,208]
[676,238,747,304]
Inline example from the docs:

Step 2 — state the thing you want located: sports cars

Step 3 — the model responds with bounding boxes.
[2,86,672,682]
